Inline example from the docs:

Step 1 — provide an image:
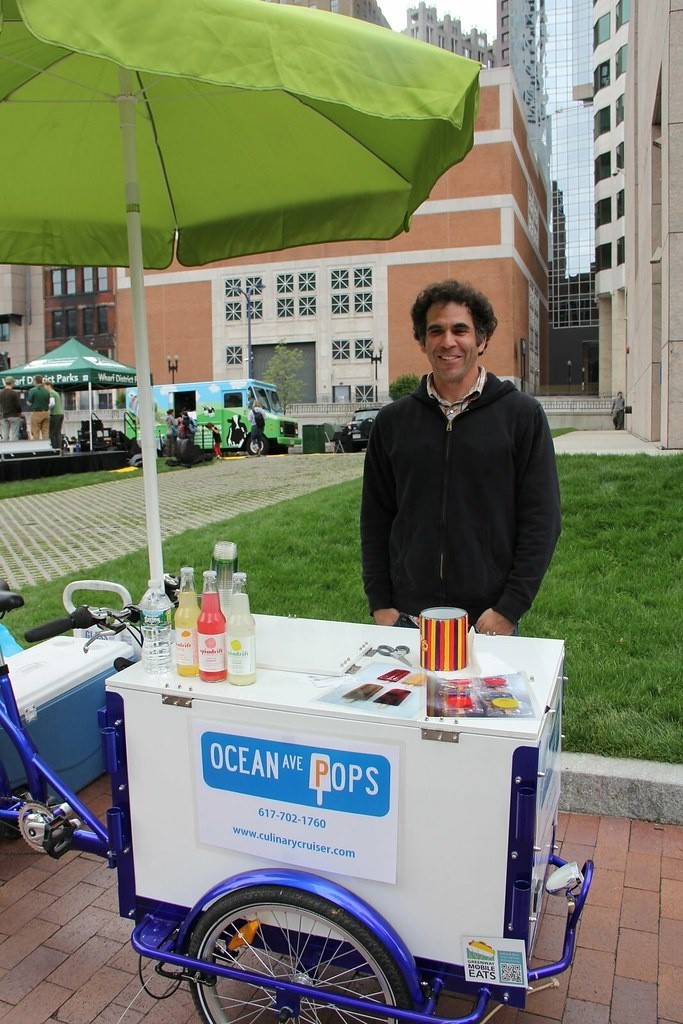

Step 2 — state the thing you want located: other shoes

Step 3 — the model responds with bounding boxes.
[217,455,220,459]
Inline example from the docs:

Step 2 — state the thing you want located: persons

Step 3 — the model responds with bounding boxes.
[248,400,268,457]
[611,392,625,430]
[0,375,64,453]
[206,423,223,459]
[166,407,196,455]
[360,280,562,640]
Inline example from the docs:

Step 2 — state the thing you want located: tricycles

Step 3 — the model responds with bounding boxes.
[1,591,596,1024]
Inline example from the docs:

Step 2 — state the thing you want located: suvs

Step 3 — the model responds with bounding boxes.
[81,427,118,451]
[345,406,385,452]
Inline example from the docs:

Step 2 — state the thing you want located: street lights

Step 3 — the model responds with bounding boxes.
[231,282,266,380]
[167,353,180,384]
[370,341,385,403]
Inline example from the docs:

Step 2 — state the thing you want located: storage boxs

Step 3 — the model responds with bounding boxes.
[0,636,137,796]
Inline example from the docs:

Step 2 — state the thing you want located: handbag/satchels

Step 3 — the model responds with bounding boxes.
[252,408,265,429]
[170,425,182,436]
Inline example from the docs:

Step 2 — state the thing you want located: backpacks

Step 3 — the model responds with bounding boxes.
[184,417,197,434]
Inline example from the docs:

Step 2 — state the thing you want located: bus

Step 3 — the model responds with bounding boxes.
[126,381,302,452]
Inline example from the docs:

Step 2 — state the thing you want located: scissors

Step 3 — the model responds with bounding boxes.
[378,646,413,667]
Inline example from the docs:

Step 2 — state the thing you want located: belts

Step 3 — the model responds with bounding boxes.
[33,409,48,412]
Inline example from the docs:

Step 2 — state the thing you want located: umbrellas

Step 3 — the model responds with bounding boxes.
[0,0,481,611]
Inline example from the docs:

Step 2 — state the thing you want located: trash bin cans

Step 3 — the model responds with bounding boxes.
[302,423,335,454]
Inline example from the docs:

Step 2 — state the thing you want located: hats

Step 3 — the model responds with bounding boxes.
[4,376,17,383]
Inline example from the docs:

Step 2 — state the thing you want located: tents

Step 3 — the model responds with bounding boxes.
[0,335,154,453]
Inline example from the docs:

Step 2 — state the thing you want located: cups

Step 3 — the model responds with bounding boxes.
[207,541,238,615]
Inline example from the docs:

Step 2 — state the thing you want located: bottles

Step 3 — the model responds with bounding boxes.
[138,580,172,675]
[225,572,257,686]
[174,566,201,677]
[196,569,228,683]
